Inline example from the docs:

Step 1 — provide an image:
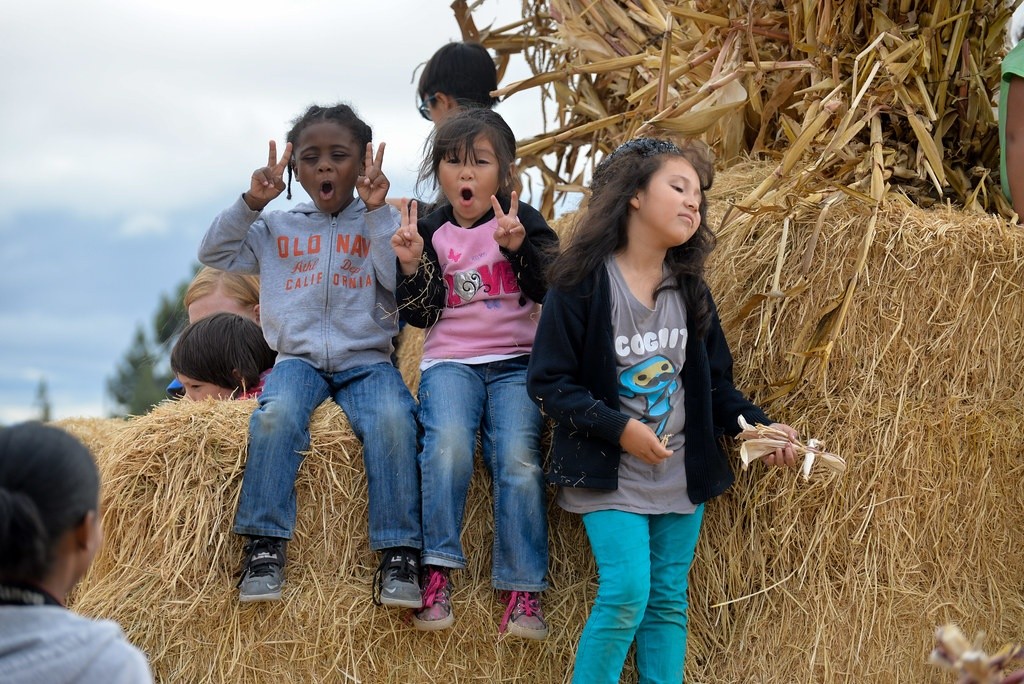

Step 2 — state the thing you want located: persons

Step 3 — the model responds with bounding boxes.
[196,98,427,610]
[0,418,156,684]
[524,136,799,683]
[993,24,1024,225]
[385,39,502,218]
[164,262,263,396]
[384,105,562,641]
[169,311,277,400]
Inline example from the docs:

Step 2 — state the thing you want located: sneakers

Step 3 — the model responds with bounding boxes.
[238,541,283,602]
[380,550,422,608]
[411,570,452,630]
[505,592,549,641]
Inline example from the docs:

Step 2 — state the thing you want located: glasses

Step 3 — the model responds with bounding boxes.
[417,95,436,122]
